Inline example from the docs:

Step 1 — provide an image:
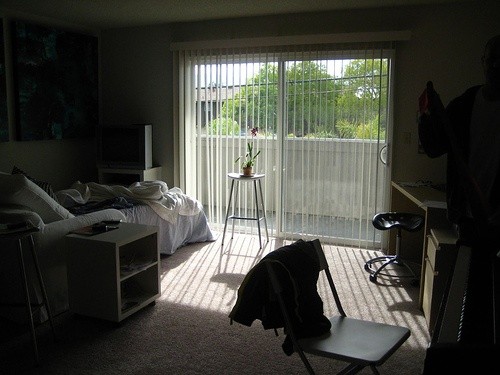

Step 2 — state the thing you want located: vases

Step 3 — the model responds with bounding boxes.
[243,167,252,176]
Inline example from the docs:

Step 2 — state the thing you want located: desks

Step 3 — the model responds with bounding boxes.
[392,180,447,310]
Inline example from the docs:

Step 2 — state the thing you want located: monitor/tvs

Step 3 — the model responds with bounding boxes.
[96,121,153,170]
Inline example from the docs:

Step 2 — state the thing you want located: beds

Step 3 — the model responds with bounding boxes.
[0,171,215,325]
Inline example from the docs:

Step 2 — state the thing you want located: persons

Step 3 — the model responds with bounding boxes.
[419,35,500,343]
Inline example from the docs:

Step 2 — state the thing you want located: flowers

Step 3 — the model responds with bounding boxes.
[235,127,261,169]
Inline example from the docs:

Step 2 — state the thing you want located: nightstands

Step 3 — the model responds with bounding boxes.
[65,223,160,326]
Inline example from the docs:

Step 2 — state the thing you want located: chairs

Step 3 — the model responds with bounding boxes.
[262,240,411,375]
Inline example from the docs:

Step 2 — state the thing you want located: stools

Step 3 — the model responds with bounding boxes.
[221,173,270,250]
[431,240,500,344]
[0,224,58,369]
[364,211,424,287]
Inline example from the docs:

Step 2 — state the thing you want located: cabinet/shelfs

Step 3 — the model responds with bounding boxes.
[97,166,162,184]
[423,227,458,334]
